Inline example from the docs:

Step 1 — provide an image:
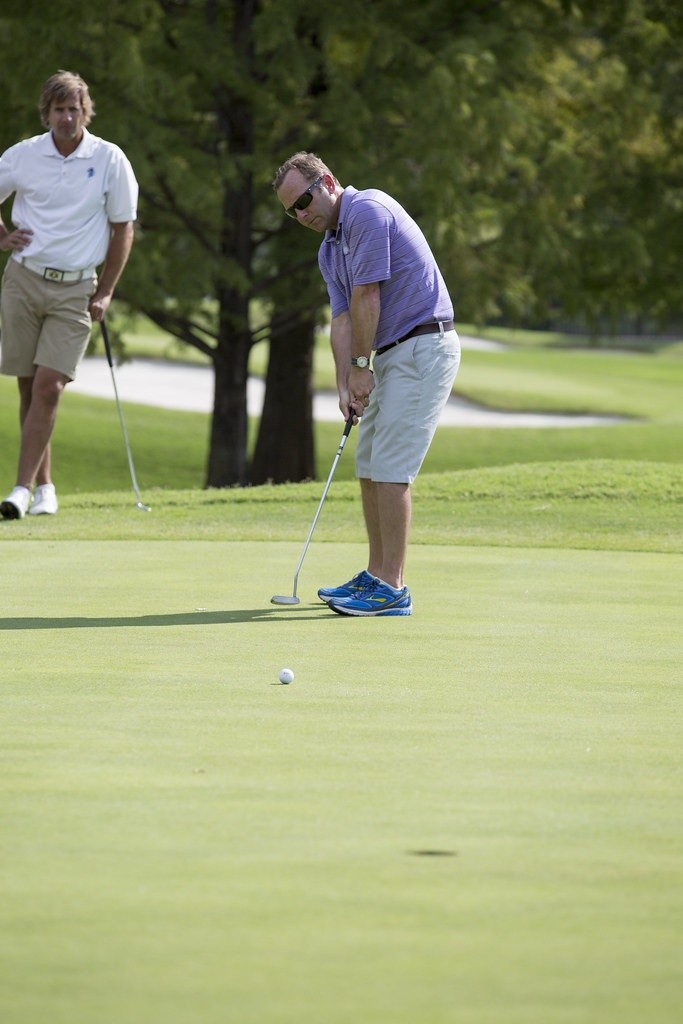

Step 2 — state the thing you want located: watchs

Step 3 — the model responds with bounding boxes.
[351,356,369,369]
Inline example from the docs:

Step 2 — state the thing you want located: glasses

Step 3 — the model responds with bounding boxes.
[284,176,325,220]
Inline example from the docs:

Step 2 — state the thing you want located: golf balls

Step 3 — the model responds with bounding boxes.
[279,668,294,684]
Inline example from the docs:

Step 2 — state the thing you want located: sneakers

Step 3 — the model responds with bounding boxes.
[327,577,414,618]
[29,483,60,515]
[0,484,33,520]
[316,569,376,603]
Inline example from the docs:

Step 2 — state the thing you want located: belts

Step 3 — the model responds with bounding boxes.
[10,249,96,283]
[375,320,455,355]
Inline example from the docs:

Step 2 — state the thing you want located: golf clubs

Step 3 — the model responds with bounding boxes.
[99,317,152,512]
[270,369,374,605]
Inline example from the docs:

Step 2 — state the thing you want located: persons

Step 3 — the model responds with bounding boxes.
[0,72,139,520]
[272,152,461,617]
